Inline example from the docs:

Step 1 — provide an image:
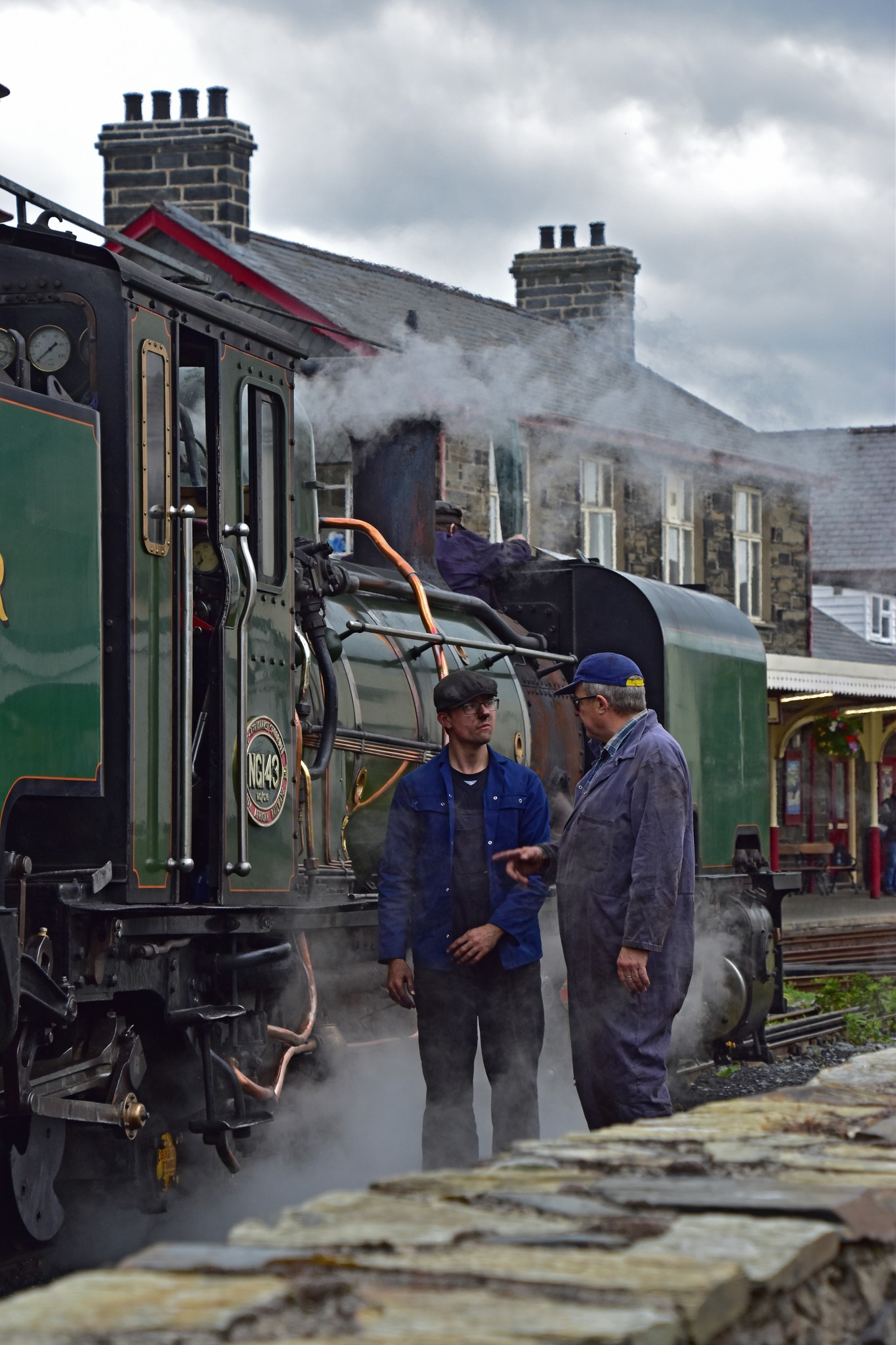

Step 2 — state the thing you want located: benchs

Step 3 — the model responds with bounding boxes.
[777,843,859,896]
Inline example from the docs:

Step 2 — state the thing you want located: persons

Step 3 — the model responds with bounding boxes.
[378,670,553,1170]
[492,652,695,1132]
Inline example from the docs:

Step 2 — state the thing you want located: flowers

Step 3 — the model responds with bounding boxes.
[807,709,863,758]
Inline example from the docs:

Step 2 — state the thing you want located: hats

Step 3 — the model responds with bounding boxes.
[434,670,498,713]
[552,653,645,697]
[435,498,466,529]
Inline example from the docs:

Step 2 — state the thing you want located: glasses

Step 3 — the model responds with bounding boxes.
[572,694,610,714]
[458,697,500,715]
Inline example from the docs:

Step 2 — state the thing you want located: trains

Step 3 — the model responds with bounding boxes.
[1,177,806,1251]
